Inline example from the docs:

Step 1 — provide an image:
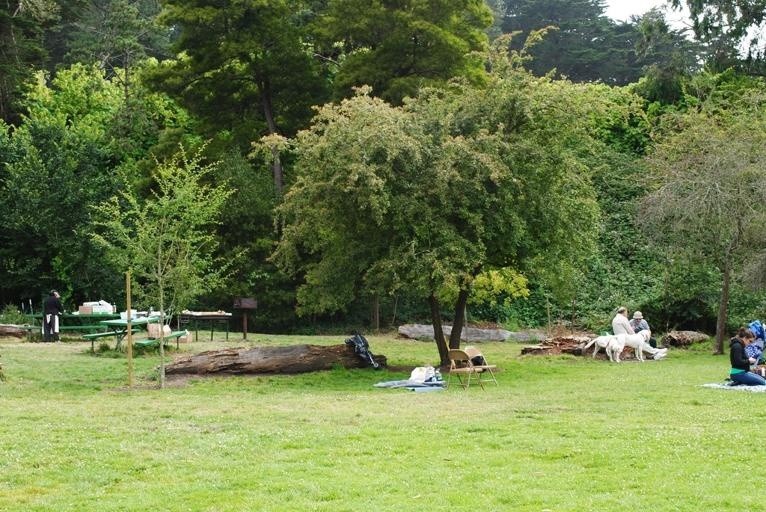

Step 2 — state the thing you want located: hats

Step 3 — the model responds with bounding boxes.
[633,311,643,319]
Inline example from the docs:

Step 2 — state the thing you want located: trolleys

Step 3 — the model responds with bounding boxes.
[344,331,380,370]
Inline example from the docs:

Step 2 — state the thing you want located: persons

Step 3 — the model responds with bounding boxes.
[727,325,766,386]
[41,289,64,342]
[612,307,667,361]
[742,321,766,364]
[627,311,657,359]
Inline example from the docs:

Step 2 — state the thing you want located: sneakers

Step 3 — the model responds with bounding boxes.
[646,348,668,361]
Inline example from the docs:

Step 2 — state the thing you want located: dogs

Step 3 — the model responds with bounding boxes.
[605,329,652,363]
[584,335,613,359]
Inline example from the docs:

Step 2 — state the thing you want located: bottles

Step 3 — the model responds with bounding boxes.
[113,302,116,314]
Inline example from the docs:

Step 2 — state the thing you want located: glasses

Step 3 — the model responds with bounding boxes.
[746,337,753,343]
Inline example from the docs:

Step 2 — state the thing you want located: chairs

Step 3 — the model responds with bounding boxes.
[447,343,498,391]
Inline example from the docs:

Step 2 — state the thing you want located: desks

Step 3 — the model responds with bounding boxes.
[19,309,233,355]
